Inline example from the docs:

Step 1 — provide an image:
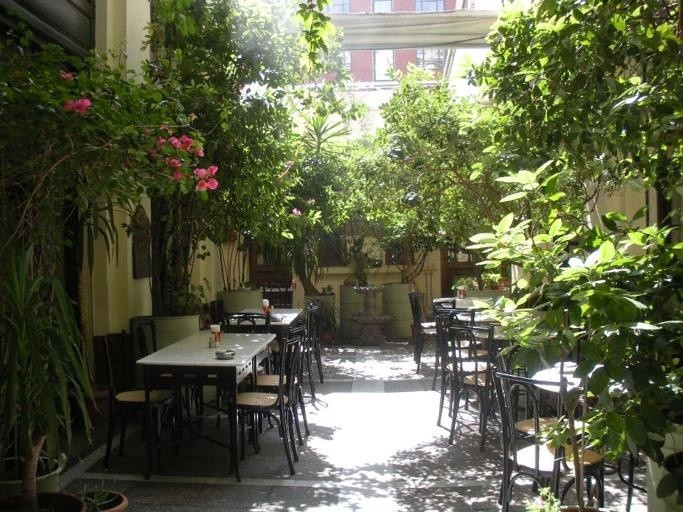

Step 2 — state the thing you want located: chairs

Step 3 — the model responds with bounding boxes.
[240,325,311,445]
[226,336,302,483]
[133,319,205,442]
[102,335,179,479]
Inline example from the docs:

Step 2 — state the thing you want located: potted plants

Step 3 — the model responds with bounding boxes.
[452,157,683,512]
[0,230,127,512]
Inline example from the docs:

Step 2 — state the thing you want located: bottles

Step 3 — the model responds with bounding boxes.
[263,306,275,314]
[208,332,224,347]
[457,287,466,299]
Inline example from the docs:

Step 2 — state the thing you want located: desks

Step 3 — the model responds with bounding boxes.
[136,329,279,485]
